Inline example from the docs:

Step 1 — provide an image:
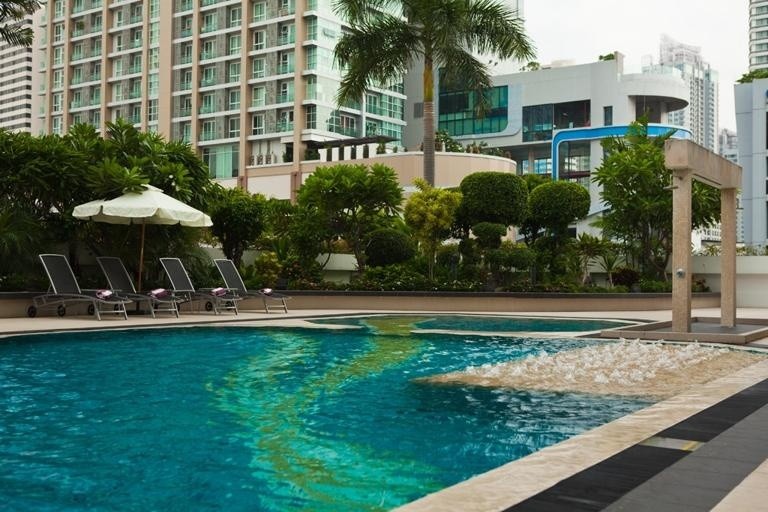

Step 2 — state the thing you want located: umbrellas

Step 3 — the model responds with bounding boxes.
[71,181,213,309]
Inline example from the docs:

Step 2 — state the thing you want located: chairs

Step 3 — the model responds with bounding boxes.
[28,254,293,322]
